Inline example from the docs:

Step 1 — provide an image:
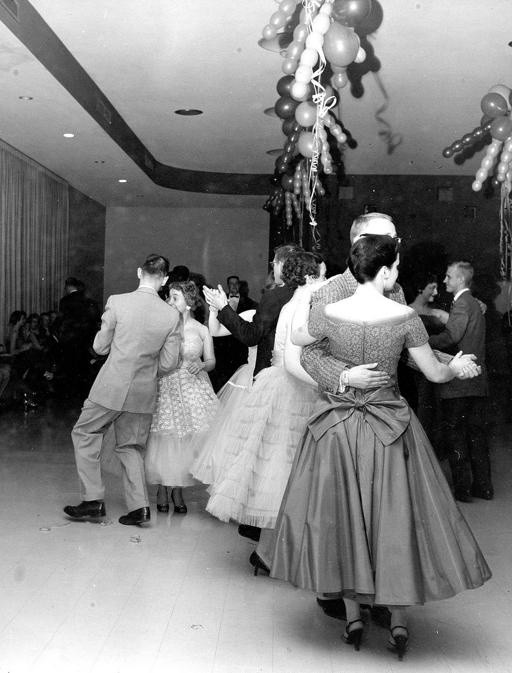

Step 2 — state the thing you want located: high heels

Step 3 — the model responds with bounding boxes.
[341,619,366,651]
[387,626,409,660]
[249,550,270,576]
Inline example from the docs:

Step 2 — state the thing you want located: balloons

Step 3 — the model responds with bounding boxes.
[443,84,512,193]
[262,0,371,227]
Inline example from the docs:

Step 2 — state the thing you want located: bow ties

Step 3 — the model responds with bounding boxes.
[230,295,239,298]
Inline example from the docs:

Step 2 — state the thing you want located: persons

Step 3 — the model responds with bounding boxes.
[61,255,216,524]
[1,278,99,408]
[191,213,494,662]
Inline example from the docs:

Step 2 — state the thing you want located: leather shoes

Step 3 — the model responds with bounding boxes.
[455,488,470,502]
[470,487,493,500]
[157,490,169,512]
[360,603,391,629]
[64,501,105,518]
[317,597,347,621]
[171,490,187,513]
[119,508,150,524]
